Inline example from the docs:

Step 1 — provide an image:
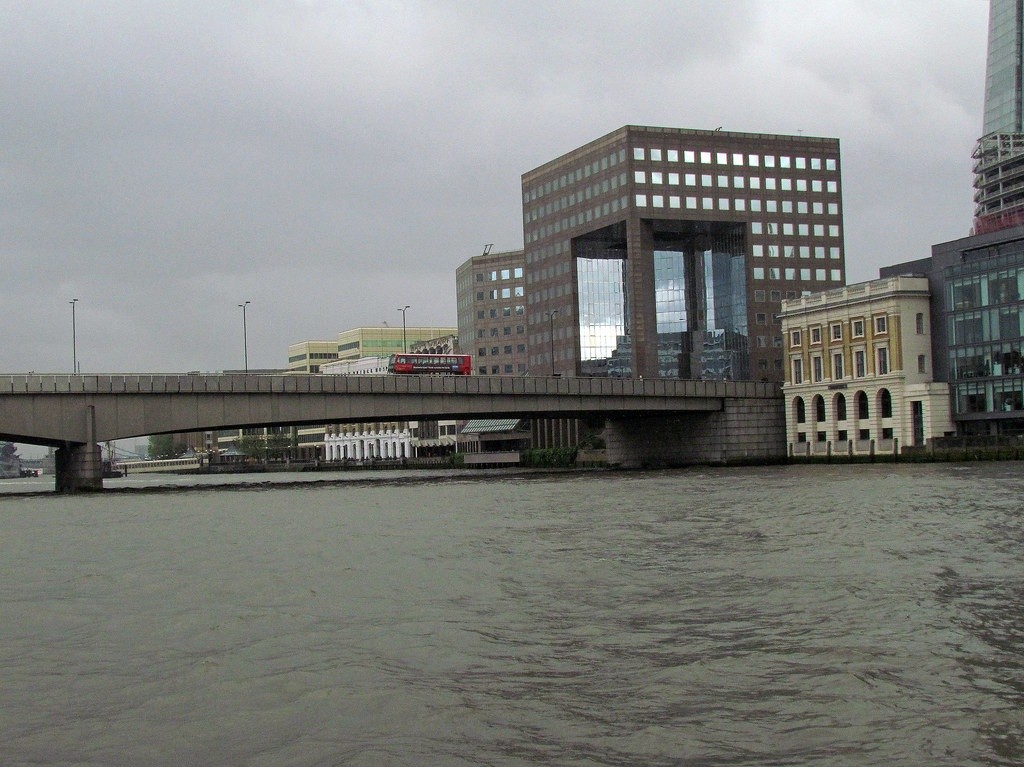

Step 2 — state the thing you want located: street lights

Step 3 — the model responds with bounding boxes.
[550,310,562,375]
[69,298,78,374]
[237,301,250,372]
[397,305,411,354]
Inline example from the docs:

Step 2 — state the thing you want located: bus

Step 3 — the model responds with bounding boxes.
[387,352,473,375]
